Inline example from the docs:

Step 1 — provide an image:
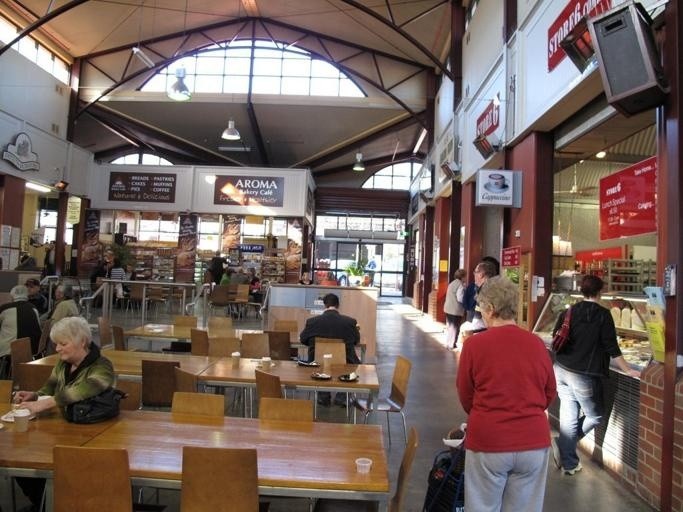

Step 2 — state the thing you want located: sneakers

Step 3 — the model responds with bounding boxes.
[564,462,583,475]
[551,437,562,469]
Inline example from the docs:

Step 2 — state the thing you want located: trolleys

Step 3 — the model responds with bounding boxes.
[422,426,467,507]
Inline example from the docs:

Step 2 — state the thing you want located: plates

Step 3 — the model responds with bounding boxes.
[338,374,359,382]
[484,181,510,194]
[311,372,332,380]
[1,412,37,423]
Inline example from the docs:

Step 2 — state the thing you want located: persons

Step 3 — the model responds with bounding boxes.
[456,276,556,512]
[12,315,117,508]
[0,240,136,379]
[550,274,641,476]
[443,256,500,352]
[297,272,314,286]
[211,251,260,318]
[300,293,360,407]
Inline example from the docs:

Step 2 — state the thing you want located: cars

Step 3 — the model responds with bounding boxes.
[335,262,402,292]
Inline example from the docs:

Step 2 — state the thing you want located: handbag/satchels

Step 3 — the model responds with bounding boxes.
[73,389,122,424]
[552,326,570,353]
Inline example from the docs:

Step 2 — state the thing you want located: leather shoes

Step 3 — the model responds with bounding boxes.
[317,397,331,406]
[336,397,356,405]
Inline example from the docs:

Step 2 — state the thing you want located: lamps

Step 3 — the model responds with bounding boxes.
[167,0,191,104]
[352,141,365,172]
[221,0,241,140]
[132,0,155,69]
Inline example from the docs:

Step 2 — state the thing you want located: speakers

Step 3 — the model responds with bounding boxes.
[588,1,666,119]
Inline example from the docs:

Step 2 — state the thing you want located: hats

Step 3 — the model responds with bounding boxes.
[24,278,39,286]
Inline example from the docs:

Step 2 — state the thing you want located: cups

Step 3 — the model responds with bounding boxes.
[12,408,31,433]
[487,174,511,189]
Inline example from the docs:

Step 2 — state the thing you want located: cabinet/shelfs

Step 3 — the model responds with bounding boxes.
[607,258,657,297]
